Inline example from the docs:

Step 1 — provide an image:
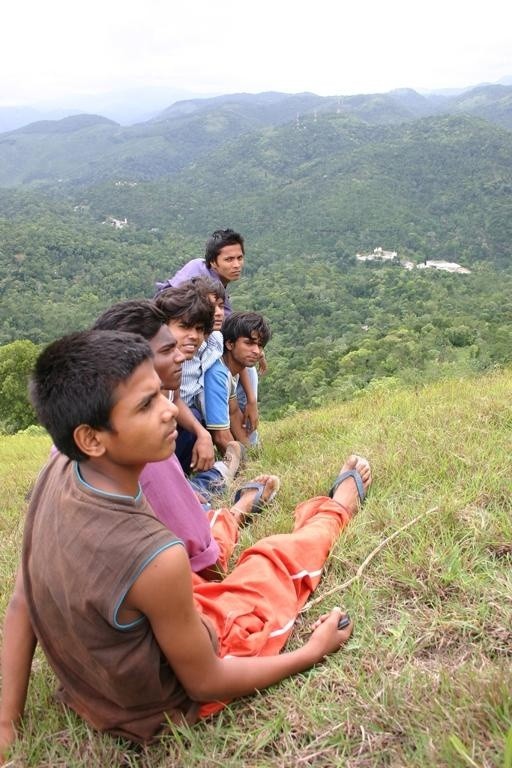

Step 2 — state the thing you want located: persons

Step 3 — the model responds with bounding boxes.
[152,285,215,477]
[148,228,267,377]
[180,276,259,436]
[93,300,241,582]
[196,311,269,458]
[0,329,373,767]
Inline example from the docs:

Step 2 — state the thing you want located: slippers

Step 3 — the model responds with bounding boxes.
[328,454,372,518]
[231,473,282,524]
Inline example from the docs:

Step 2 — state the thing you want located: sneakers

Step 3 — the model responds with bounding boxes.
[222,440,243,482]
